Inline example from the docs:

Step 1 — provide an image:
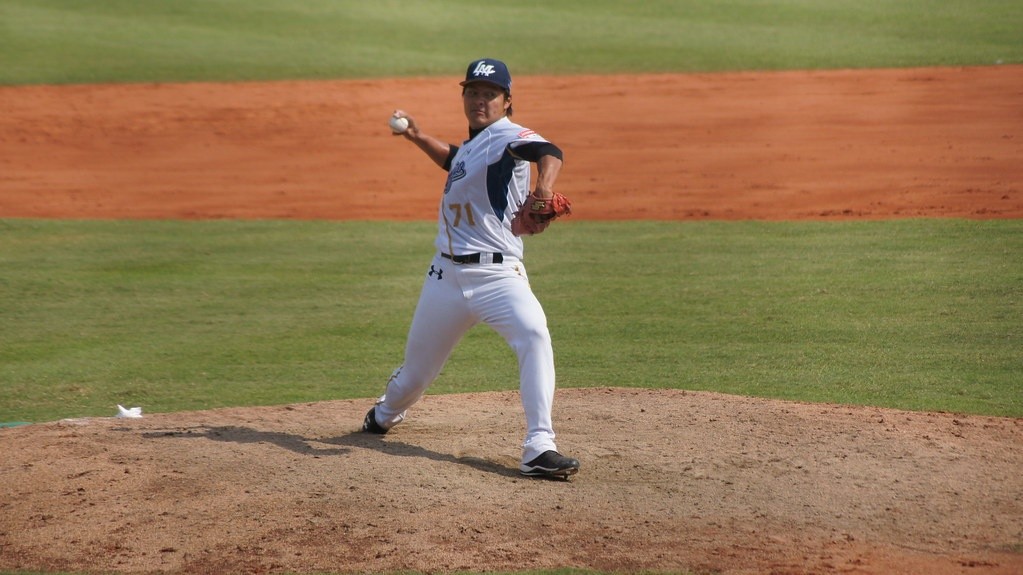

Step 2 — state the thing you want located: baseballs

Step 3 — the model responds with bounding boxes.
[389,116,409,134]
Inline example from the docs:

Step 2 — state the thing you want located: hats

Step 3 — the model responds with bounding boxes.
[459,58,511,94]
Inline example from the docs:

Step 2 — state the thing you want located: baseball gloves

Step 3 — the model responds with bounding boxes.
[510,192,572,238]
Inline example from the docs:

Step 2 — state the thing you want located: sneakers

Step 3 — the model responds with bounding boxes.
[363,407,389,434]
[520,450,580,481]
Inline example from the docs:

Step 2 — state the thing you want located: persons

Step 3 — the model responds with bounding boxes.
[361,58,581,481]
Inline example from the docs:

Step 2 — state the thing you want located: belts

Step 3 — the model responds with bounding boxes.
[441,252,503,263]
